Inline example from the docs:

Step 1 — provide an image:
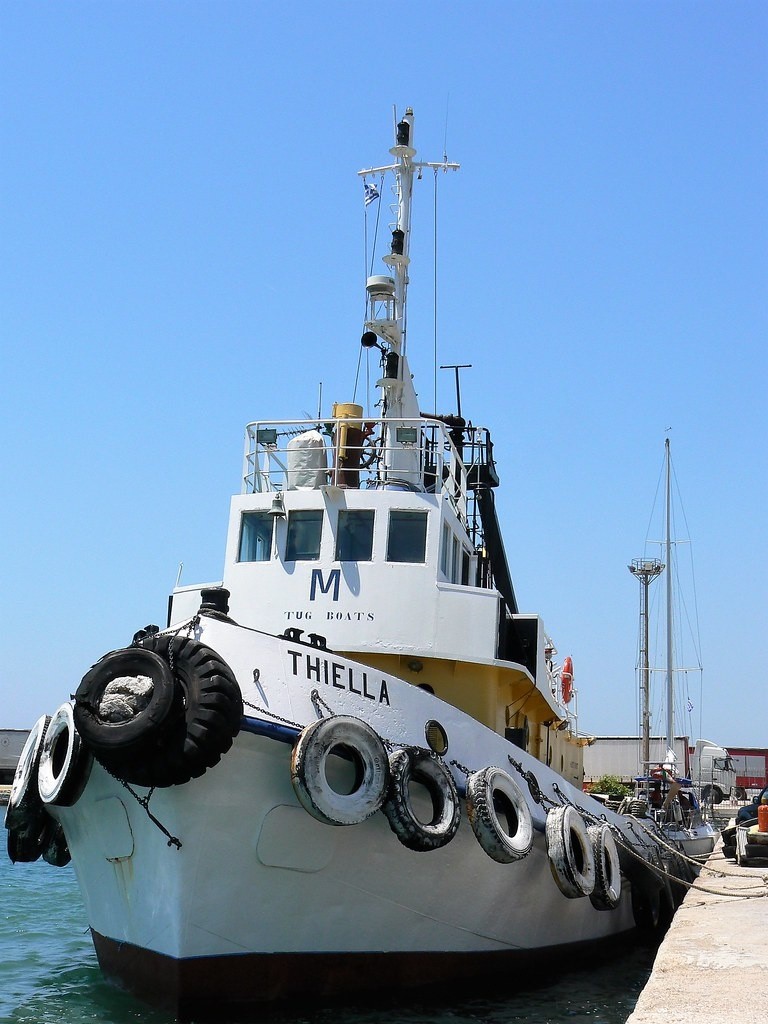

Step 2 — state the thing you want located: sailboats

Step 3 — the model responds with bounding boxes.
[615,425,721,876]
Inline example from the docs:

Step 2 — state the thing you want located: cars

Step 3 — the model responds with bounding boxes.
[735,786,768,824]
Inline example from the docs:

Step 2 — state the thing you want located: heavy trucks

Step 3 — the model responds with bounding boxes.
[576,734,737,809]
[685,745,767,799]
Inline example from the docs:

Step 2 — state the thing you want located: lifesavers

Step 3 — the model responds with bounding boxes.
[561,657,573,703]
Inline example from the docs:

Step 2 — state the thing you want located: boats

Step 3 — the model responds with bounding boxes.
[0,104,677,1023]
